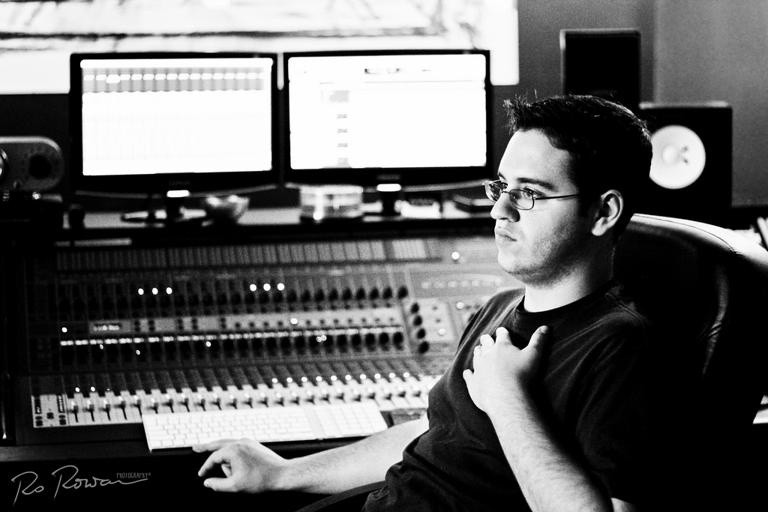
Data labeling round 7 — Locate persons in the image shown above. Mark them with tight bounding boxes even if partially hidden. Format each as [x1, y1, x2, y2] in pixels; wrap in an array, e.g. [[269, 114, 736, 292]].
[[188, 83, 684, 511]]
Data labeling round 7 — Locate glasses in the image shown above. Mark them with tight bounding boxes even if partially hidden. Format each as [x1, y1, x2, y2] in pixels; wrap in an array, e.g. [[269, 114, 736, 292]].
[[483, 179, 578, 210]]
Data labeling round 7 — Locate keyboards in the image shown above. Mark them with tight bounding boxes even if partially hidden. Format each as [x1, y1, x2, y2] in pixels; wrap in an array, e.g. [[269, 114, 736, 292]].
[[140, 400, 389, 453]]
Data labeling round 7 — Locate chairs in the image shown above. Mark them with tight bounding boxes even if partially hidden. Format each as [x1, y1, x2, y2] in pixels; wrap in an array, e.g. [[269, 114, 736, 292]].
[[292, 203, 767, 512]]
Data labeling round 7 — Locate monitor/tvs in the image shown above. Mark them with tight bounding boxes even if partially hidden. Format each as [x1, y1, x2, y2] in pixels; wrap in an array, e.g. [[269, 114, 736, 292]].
[[282, 49, 492, 218], [69, 52, 279, 224]]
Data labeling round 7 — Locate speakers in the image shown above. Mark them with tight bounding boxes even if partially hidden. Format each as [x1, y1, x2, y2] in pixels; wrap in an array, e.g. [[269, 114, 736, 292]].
[[559, 28, 642, 107], [635, 100, 734, 223]]
